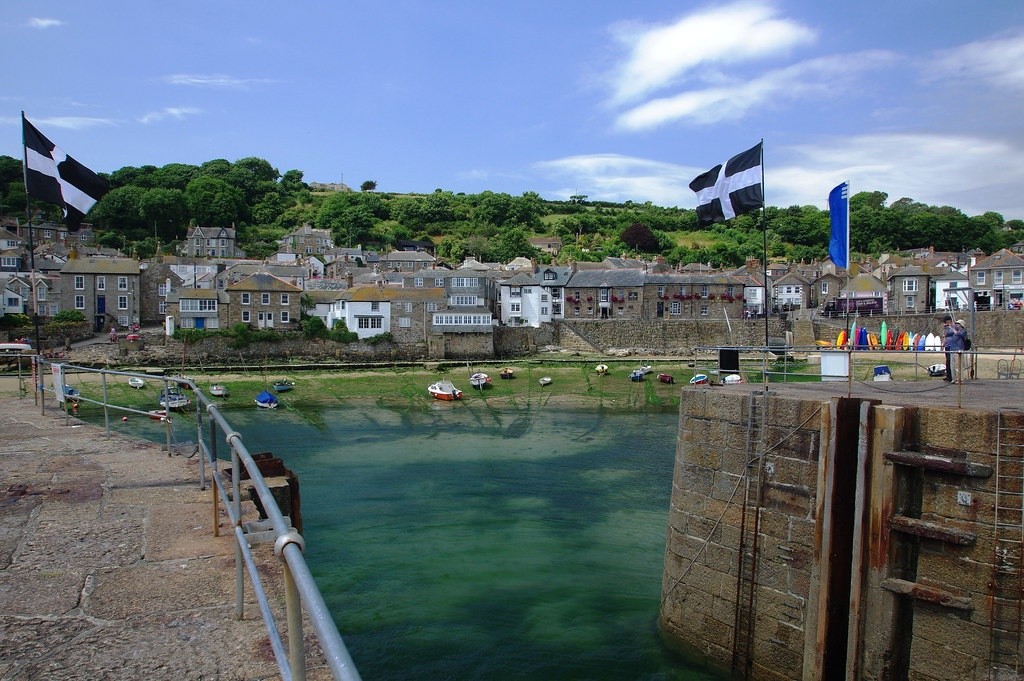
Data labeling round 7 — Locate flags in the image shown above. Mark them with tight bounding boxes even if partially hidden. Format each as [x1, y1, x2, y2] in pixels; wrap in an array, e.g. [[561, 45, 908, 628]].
[[826, 182, 848, 268], [688, 141, 765, 227], [23, 117, 109, 233]]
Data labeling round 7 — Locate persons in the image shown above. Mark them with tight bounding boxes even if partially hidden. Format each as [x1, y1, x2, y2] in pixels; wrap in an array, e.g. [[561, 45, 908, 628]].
[[947, 319, 969, 384], [941, 316, 955, 381]]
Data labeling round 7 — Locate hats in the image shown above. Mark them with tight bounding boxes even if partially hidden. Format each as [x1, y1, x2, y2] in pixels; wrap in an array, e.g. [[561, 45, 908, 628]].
[[954, 320, 966, 329]]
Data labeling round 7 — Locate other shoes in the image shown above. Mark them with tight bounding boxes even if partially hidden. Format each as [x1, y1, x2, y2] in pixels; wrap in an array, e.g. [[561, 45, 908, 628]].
[[955, 381, 963, 384], [950, 380, 956, 384], [943, 378, 951, 381]]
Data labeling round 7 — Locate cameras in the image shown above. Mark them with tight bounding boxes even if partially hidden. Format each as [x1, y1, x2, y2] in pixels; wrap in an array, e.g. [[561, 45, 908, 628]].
[[945, 324, 949, 326]]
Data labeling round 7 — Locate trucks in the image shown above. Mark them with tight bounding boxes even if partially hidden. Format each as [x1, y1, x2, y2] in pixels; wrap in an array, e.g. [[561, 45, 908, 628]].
[[822, 295, 884, 318]]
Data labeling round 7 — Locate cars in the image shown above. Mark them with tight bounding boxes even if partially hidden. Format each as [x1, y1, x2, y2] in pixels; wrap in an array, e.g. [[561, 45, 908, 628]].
[[0, 343, 31, 355]]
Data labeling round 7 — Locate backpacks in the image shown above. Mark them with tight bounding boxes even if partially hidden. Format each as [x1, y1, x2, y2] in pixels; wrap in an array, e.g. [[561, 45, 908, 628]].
[[961, 337, 971, 350]]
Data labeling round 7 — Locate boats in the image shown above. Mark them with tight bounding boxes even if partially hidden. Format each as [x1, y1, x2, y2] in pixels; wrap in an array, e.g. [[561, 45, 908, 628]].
[[174, 373, 195, 389], [640, 366, 652, 374], [272, 379, 295, 392], [594, 364, 608, 375], [209, 384, 228, 397], [428, 379, 463, 401], [62, 384, 82, 401], [659, 374, 676, 384], [720, 374, 745, 385], [631, 369, 644, 381], [539, 376, 551, 386], [469, 372, 488, 389], [59, 398, 78, 411], [255, 389, 278, 408], [159, 393, 188, 413], [500, 368, 514, 379], [873, 365, 893, 382], [927, 363, 946, 376], [148, 410, 167, 419], [128, 376, 144, 389], [689, 374, 707, 385]]
